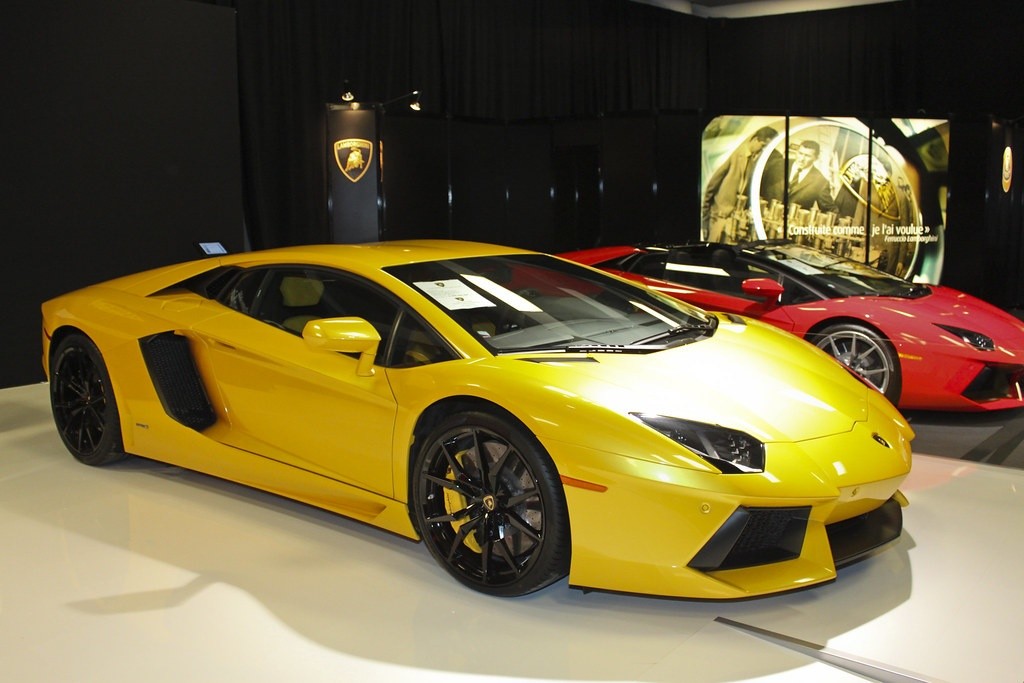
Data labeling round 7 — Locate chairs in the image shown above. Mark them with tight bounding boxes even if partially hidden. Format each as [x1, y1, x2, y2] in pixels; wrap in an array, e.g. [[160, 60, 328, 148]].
[[711, 250, 741, 293], [279, 278, 324, 334], [666, 252, 701, 288]]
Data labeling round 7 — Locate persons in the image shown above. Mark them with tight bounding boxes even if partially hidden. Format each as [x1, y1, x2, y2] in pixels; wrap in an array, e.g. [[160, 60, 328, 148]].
[[703, 124, 917, 278]]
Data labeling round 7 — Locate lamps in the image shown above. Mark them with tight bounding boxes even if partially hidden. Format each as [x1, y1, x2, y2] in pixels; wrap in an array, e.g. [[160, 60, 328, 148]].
[[374, 90, 422, 111], [340, 75, 354, 100]]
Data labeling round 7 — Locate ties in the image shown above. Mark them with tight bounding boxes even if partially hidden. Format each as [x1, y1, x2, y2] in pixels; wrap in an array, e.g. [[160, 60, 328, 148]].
[[788, 168, 802, 194]]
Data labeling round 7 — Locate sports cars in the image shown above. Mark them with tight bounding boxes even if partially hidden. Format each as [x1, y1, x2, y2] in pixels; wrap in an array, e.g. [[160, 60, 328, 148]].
[[42, 240, 915, 599], [483, 241, 1024, 412]]
